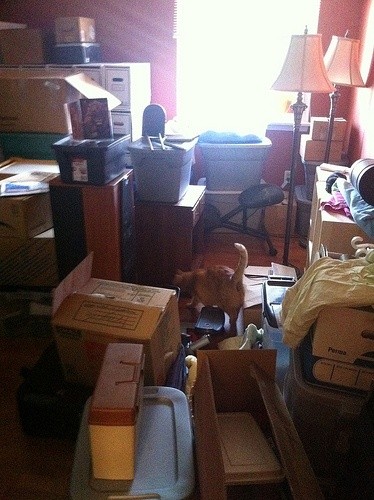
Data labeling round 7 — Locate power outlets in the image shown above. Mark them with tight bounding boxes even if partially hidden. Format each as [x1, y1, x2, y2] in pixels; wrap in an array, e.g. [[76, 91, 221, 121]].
[[284, 171, 291, 184]]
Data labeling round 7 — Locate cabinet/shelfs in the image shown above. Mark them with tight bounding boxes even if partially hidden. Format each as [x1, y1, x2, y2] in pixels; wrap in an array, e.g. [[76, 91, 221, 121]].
[[0, 62, 151, 141]]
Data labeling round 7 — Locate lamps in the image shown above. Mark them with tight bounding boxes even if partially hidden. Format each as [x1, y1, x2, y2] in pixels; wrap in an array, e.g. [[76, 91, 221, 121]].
[[324, 29, 366, 163], [271, 25, 337, 280]]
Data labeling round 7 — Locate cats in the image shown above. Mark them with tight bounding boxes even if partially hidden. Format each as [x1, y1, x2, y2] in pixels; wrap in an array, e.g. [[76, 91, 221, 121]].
[[168, 242, 248, 337]]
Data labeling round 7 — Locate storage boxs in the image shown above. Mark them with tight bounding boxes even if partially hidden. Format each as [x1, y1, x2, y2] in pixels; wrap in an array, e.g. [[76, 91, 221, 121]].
[[262, 275, 297, 379], [193, 350, 325, 500], [198, 177, 266, 233], [301, 157, 346, 201], [0, 70, 123, 136], [299, 134, 342, 161], [89, 343, 145, 480], [294, 185, 312, 248], [264, 191, 297, 238], [300, 252, 374, 398], [311, 199, 374, 266], [0, 134, 206, 390], [70, 386, 195, 500], [283, 348, 365, 478], [314, 166, 335, 190], [197, 136, 272, 191], [0, 16, 100, 63], [310, 182, 332, 241], [310, 117, 347, 141]]
